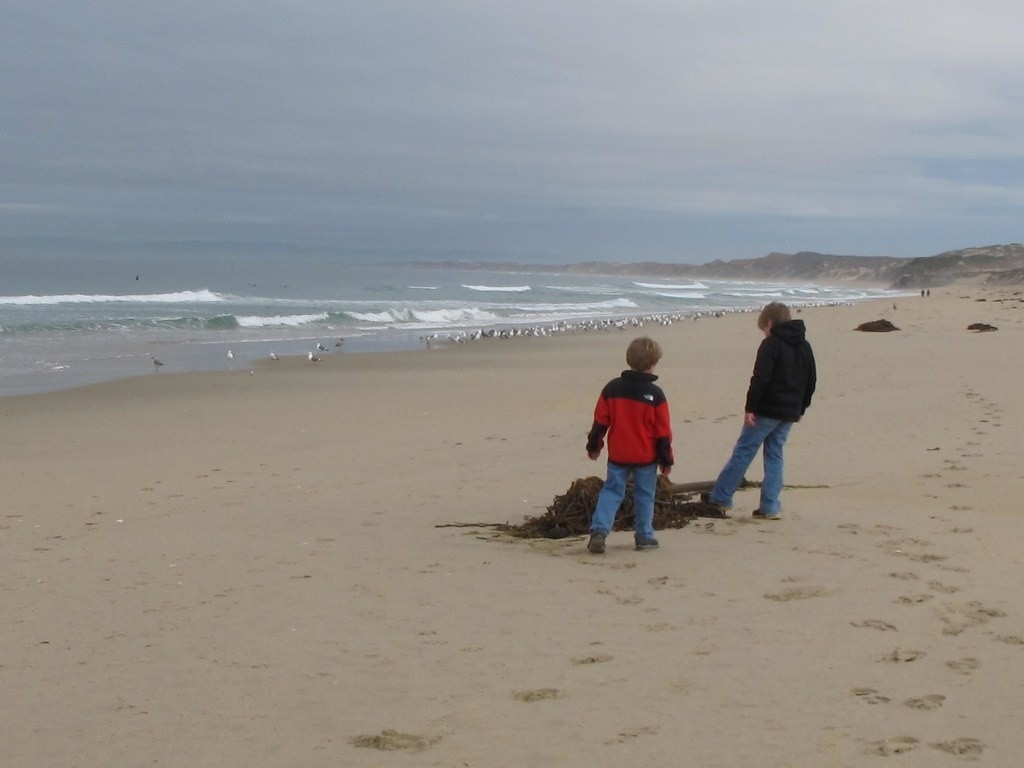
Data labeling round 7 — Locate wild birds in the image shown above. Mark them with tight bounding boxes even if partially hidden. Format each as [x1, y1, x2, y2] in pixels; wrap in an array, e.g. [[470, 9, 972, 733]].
[[151, 356, 165, 368], [268, 336, 347, 364], [225, 349, 234, 359], [410, 299, 855, 345]]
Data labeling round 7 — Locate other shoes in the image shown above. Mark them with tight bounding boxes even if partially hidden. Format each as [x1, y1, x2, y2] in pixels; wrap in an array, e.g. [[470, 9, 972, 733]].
[[634, 533, 659, 549], [753, 508, 780, 519], [586, 529, 606, 553], [695, 505, 726, 519]]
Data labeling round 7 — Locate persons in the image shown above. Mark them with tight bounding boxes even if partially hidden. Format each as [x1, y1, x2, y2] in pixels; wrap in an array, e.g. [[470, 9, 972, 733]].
[[700, 301, 817, 519], [586, 336, 674, 556]]
